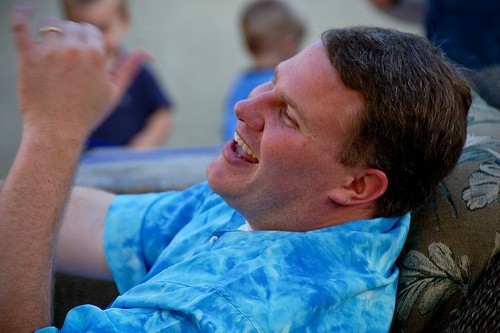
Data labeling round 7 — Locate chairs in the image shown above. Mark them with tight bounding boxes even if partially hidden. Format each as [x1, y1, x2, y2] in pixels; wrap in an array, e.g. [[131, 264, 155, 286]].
[[390, 84, 500, 333]]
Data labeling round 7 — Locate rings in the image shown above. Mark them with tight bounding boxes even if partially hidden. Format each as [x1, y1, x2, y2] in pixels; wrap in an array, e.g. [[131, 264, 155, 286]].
[[37, 27, 64, 33]]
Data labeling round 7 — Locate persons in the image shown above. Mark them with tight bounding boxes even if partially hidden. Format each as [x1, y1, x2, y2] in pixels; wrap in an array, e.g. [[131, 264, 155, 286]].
[[371, 0, 500, 168], [223, 0, 308, 144], [51, 0, 174, 154], [0, 0, 473, 333]]
